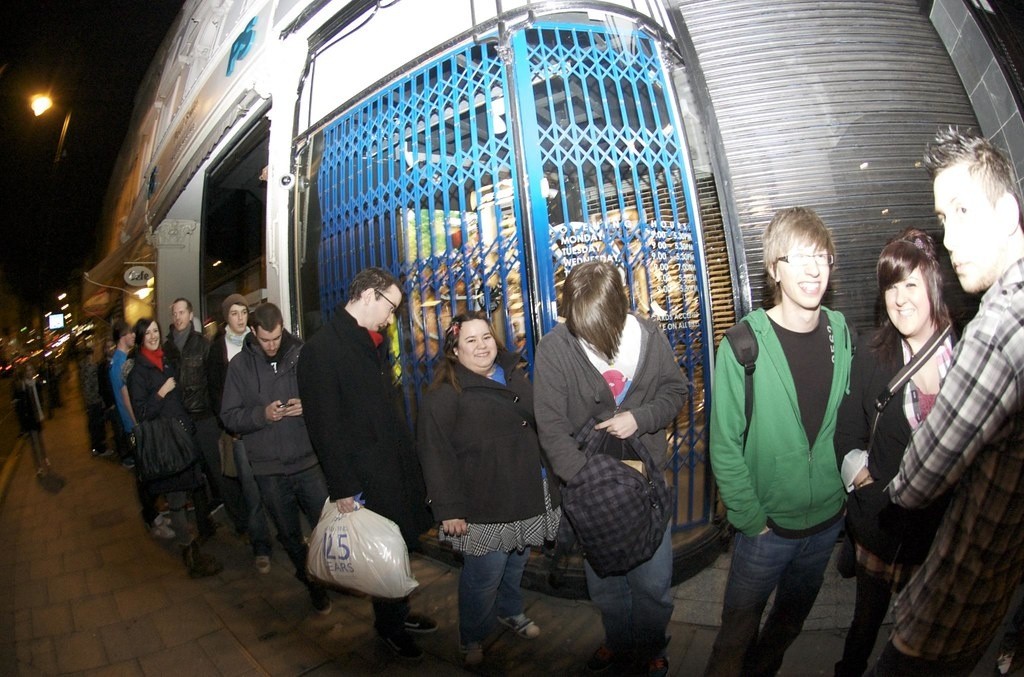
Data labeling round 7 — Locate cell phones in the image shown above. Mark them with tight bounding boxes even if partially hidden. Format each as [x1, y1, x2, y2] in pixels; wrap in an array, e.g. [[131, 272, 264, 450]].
[[280, 403, 289, 408]]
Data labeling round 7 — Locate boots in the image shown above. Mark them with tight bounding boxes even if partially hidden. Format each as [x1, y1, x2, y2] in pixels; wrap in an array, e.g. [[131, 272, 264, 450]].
[[178, 535, 223, 575]]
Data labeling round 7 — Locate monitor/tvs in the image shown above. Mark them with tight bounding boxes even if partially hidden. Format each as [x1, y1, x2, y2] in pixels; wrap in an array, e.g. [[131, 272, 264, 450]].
[[49, 314, 64, 330]]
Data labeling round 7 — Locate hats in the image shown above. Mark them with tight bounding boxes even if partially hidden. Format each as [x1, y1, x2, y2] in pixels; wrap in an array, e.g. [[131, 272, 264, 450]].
[[222, 293, 248, 322]]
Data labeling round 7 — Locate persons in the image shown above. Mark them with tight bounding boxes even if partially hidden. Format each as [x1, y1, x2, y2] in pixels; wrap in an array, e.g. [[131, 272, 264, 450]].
[[220, 303, 368, 615], [297, 267, 440, 653], [533, 259, 687, 675], [710, 207, 852, 675], [18, 363, 56, 479], [417, 311, 562, 667], [77, 124, 1024, 677], [126, 316, 226, 579]]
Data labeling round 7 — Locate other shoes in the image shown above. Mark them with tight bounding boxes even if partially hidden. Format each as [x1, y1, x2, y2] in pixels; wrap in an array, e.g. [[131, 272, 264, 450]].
[[257, 555, 271, 575], [184, 494, 195, 511], [343, 586, 366, 598], [404, 613, 439, 632], [91, 445, 114, 456], [497, 612, 541, 638], [154, 498, 170, 514], [307, 578, 333, 615], [459, 640, 482, 665], [145, 515, 176, 538], [121, 457, 135, 467], [380, 632, 425, 660]]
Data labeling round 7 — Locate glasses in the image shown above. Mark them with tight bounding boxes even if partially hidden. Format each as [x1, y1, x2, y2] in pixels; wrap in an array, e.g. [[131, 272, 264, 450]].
[[778, 253, 834, 266], [374, 289, 399, 314]]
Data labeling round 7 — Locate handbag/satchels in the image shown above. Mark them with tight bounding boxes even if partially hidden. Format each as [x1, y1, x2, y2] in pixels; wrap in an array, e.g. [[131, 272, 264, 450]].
[[306, 495, 419, 598], [558, 411, 674, 578], [134, 402, 196, 477]]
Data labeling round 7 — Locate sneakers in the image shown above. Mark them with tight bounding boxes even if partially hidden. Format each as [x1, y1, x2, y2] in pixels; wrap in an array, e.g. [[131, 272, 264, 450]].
[[586, 644, 620, 672], [648, 655, 669, 677]]
